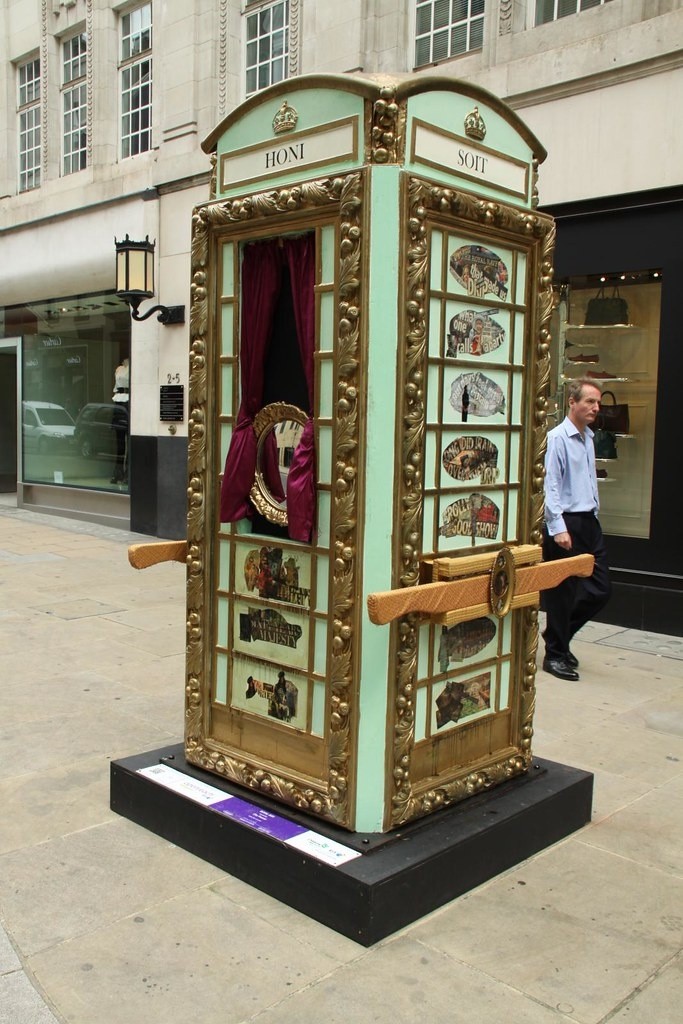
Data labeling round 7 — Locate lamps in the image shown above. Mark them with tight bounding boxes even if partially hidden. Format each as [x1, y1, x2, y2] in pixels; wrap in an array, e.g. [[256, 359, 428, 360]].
[[111, 233, 185, 327]]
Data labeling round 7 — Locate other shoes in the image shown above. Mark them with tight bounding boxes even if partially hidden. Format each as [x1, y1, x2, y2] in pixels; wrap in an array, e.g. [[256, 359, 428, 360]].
[[568, 353, 599, 364], [587, 371, 616, 378], [122, 480, 128, 486], [110, 477, 124, 484]]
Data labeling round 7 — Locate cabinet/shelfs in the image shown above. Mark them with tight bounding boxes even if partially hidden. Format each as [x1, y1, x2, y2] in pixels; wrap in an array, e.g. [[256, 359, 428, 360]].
[[566, 320, 638, 483]]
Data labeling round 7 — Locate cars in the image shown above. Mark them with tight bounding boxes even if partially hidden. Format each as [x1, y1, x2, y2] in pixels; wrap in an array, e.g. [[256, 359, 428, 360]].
[[22, 400, 76, 456], [73, 402, 130, 461]]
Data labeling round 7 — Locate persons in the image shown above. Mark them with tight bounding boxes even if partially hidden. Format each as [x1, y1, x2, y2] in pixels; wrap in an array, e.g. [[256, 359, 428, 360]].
[[111, 359, 128, 485], [539, 376, 610, 680]]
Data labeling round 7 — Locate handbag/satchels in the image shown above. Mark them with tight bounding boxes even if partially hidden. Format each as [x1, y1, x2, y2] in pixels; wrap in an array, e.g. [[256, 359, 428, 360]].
[[587, 391, 630, 434], [584, 286, 628, 326], [592, 429, 618, 459]]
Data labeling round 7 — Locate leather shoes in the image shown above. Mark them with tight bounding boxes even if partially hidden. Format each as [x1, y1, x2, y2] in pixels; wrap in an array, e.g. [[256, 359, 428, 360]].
[[565, 649, 578, 667], [543, 657, 579, 679]]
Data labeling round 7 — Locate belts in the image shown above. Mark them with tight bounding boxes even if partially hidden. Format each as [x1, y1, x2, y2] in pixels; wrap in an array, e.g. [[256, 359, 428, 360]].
[[116, 387, 129, 394]]
